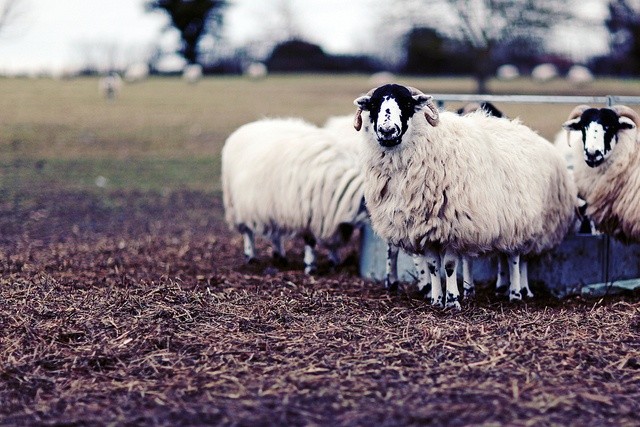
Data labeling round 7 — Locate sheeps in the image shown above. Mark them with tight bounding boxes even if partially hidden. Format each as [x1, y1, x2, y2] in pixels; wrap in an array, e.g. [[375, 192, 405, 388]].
[[383, 98, 509, 300], [219, 97, 444, 276], [352, 81, 575, 310], [553, 103, 640, 248]]
